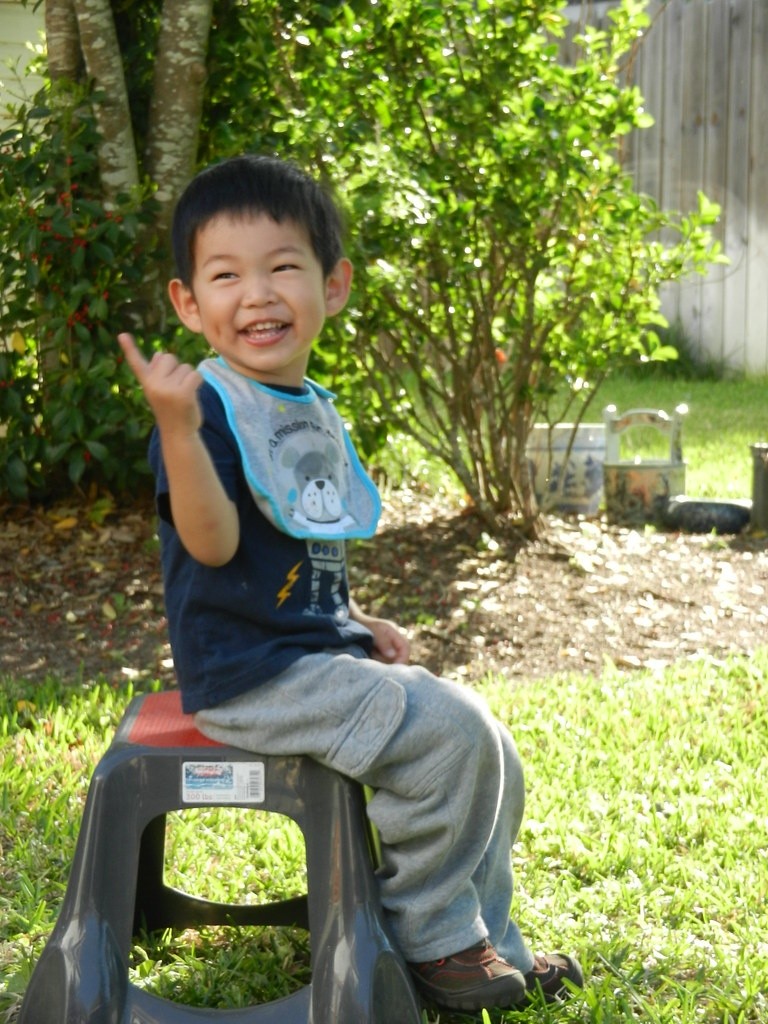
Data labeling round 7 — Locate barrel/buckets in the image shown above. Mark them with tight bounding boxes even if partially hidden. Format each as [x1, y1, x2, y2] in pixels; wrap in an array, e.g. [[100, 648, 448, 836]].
[[601, 402, 688, 526]]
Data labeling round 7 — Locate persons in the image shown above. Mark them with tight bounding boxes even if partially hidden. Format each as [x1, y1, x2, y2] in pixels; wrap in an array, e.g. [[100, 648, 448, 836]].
[[117, 159, 583, 1012]]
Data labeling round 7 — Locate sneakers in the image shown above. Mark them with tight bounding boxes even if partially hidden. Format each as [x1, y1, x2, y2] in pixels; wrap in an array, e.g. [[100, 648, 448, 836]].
[[509, 954, 583, 1008], [408, 936, 526, 1009]]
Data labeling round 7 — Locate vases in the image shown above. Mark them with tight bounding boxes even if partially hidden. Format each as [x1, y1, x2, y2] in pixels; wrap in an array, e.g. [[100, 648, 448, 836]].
[[531, 425, 607, 510]]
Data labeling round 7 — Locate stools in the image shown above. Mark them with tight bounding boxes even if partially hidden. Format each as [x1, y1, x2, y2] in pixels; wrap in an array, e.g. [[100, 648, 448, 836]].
[[16, 691, 427, 1023]]
